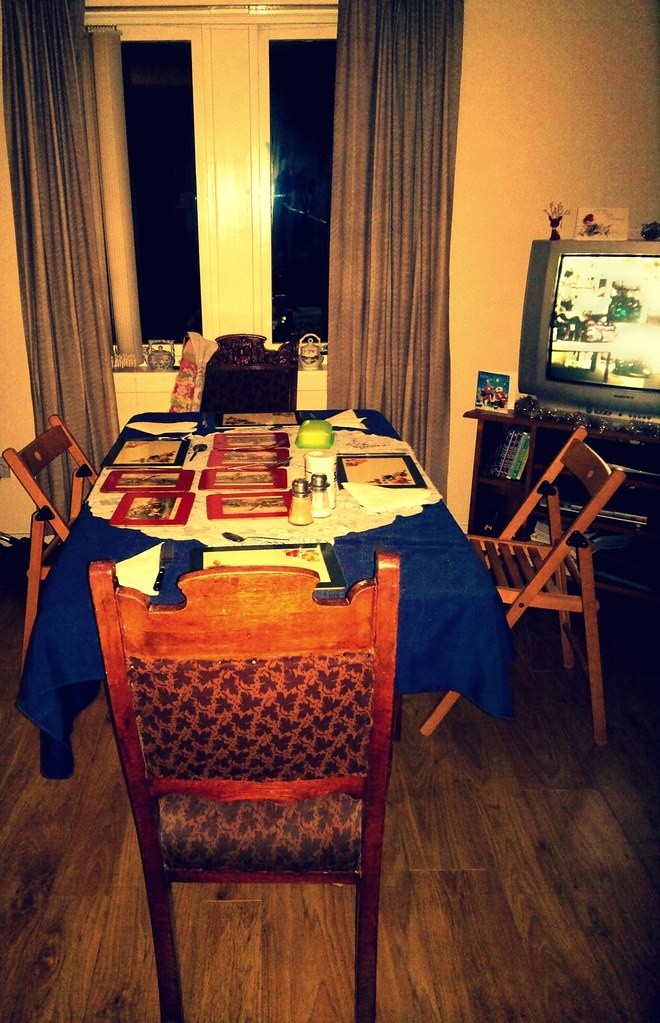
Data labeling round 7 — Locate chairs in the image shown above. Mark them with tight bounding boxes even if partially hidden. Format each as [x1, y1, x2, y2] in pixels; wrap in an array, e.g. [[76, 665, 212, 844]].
[[89, 550, 402, 1023], [420, 423, 624, 744], [184, 333, 298, 414], [2, 413, 98, 676]]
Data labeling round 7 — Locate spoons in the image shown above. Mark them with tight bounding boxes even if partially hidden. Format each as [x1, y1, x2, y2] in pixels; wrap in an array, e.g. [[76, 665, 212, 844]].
[[189, 444, 207, 462], [218, 439, 287, 449], [216, 424, 284, 430], [227, 456, 293, 469], [223, 532, 290, 542]]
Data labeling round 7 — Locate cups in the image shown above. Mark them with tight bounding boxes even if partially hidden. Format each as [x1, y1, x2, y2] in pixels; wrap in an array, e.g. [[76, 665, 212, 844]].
[[304, 449, 337, 509]]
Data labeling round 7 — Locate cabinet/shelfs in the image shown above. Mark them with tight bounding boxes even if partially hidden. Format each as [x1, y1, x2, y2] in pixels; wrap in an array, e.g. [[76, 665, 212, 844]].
[[465, 410, 660, 622]]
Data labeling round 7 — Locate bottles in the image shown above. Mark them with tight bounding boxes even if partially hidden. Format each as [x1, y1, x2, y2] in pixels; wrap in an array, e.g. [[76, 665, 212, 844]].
[[288, 475, 331, 525]]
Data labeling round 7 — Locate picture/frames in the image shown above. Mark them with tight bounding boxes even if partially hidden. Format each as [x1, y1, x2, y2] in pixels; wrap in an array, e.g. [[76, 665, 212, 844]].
[[207, 448, 289, 468], [194, 542, 348, 591], [198, 468, 287, 491], [335, 455, 427, 489], [101, 469, 195, 493], [205, 492, 292, 520], [109, 490, 195, 527], [101, 438, 191, 468], [213, 432, 291, 448]]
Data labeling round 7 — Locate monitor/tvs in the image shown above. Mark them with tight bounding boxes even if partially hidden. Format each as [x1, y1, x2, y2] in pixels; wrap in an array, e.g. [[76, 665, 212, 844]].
[[519, 238, 660, 427]]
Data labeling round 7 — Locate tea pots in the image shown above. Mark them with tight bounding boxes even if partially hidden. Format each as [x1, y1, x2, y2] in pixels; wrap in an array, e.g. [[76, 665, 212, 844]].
[[143, 340, 176, 370], [298, 333, 324, 370]]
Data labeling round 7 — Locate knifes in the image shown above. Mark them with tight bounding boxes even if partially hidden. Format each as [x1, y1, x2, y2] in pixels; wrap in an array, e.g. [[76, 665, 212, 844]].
[[336, 453, 406, 456]]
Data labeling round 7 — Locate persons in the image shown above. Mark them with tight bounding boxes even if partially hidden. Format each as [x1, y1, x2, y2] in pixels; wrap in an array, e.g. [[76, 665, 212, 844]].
[[484, 380, 493, 404], [578, 214, 599, 238], [560, 269, 579, 313], [492, 386, 507, 411]]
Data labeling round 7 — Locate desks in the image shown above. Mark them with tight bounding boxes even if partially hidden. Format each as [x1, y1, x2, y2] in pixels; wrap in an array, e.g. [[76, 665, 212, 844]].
[[14, 410, 518, 781]]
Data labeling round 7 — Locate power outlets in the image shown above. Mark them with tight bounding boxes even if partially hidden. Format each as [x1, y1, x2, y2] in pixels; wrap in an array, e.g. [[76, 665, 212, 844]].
[[0, 457, 11, 479]]
